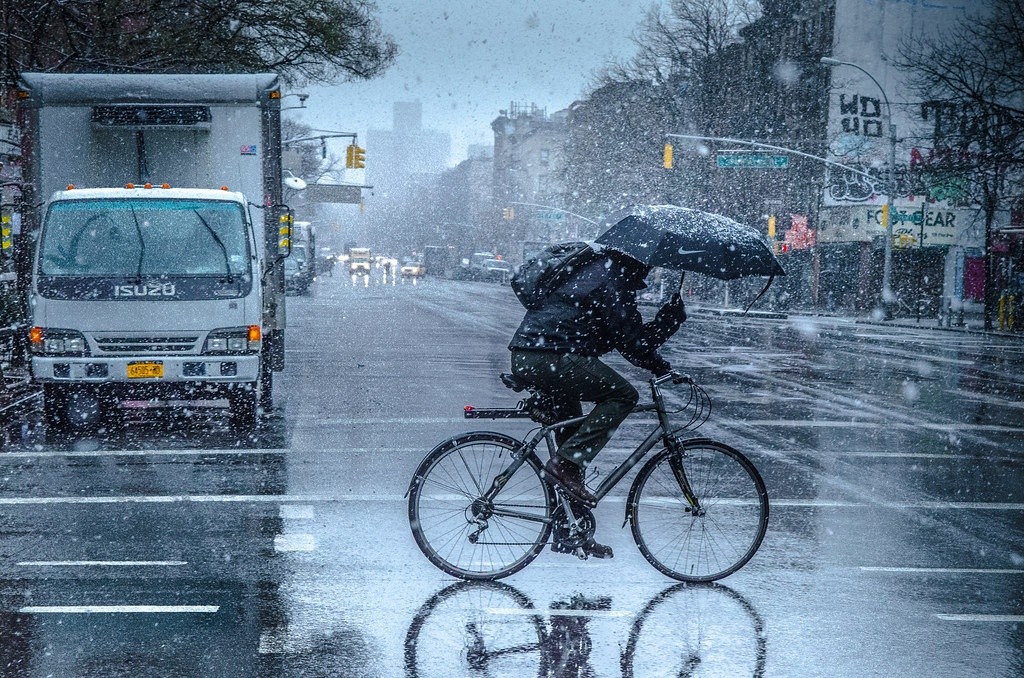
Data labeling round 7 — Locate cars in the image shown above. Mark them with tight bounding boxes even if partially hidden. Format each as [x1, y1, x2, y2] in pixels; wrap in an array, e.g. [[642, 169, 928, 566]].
[[375, 243, 522, 287], [283, 219, 351, 297], [401, 261, 425, 278]]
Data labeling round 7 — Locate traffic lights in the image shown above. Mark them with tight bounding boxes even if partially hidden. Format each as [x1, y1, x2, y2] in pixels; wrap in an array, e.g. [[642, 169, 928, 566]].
[[503, 207, 509, 219], [354, 145, 366, 168]]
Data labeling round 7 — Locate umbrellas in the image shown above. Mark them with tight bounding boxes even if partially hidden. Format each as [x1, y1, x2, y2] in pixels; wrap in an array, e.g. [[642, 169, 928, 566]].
[[594, 206, 786, 303]]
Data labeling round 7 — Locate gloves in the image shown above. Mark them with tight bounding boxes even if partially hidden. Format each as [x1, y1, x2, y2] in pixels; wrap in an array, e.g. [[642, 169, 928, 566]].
[[656, 293, 687, 324], [651, 360, 672, 378]]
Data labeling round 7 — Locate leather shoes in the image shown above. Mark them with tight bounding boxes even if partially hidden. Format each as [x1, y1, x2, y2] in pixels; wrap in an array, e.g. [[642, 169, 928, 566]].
[[540, 454, 597, 508], [551, 528, 613, 559]]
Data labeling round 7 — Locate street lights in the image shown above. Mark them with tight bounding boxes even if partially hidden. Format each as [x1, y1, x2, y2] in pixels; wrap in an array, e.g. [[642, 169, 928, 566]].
[[821, 55, 895, 321]]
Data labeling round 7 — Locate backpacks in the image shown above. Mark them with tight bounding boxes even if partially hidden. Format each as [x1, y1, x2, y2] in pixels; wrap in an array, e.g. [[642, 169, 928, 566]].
[[510, 242, 606, 310]]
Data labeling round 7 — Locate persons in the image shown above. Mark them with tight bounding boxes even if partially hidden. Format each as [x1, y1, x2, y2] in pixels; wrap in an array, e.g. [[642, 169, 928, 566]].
[[385, 262, 390, 274], [326, 256, 334, 275], [996, 250, 1019, 332], [508, 245, 687, 559]]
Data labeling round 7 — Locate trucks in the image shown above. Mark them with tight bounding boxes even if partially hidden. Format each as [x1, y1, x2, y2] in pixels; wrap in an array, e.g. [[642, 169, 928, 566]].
[[0, 71, 294, 432], [349, 246, 373, 274]]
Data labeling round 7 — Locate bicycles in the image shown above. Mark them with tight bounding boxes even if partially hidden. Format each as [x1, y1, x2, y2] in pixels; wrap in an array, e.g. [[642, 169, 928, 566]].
[[403, 365, 769, 583], [883, 279, 939, 320]]
